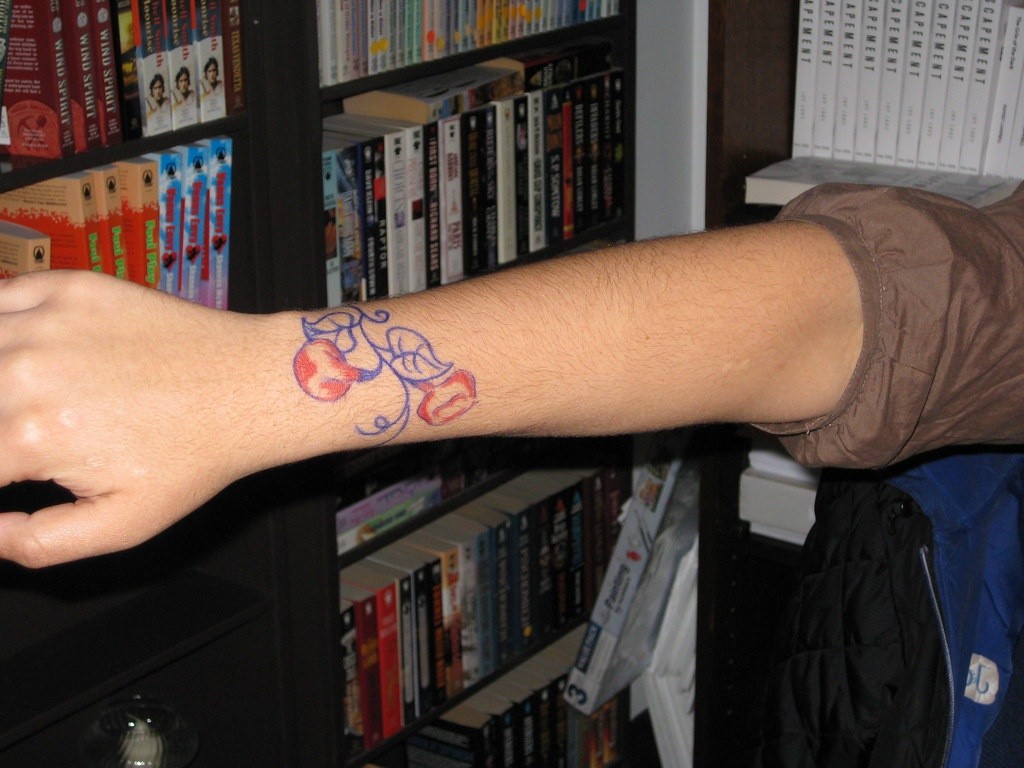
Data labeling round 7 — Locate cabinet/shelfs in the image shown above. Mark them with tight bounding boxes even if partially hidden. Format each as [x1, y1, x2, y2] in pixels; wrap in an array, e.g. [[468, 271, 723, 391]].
[[0, 0, 818, 768]]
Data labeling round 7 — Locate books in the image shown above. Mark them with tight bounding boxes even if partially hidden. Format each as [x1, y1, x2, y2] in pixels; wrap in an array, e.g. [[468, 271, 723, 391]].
[[0, 1, 1024, 768]]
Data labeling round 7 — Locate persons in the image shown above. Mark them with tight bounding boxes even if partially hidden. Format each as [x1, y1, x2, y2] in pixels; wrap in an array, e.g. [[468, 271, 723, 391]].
[[202, 57, 223, 95], [142, 73, 168, 112], [0, 181, 1024, 567], [171, 67, 195, 103]]
